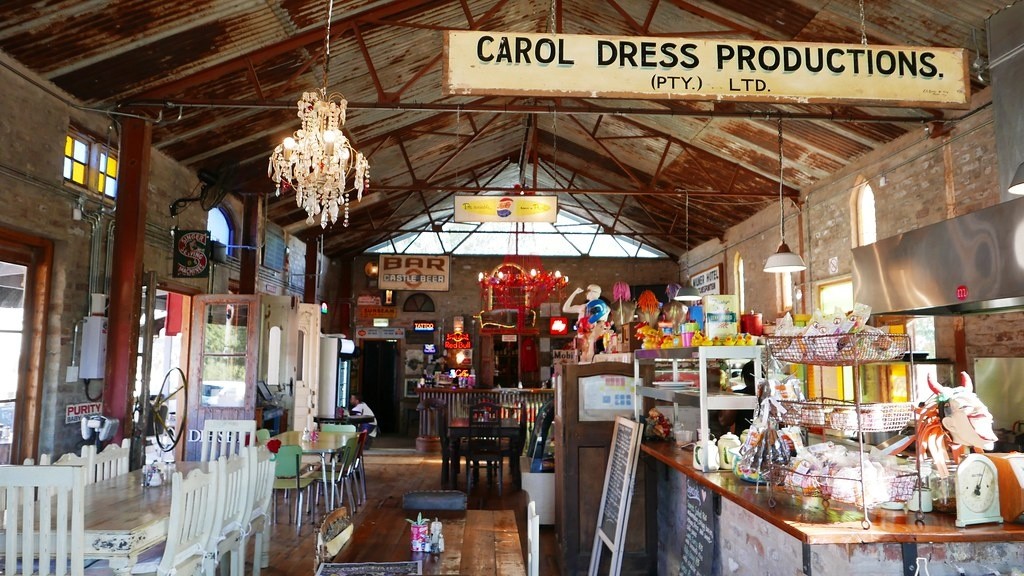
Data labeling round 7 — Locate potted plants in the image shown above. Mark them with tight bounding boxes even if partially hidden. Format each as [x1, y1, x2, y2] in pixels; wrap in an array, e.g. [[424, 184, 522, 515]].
[[404, 512, 430, 552]]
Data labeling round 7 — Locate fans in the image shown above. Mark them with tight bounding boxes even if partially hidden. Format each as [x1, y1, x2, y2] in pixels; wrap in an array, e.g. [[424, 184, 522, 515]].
[[169, 156, 241, 219]]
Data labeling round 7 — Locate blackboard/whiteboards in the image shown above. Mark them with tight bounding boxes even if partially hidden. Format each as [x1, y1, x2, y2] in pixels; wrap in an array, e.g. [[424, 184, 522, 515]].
[[596, 416, 639, 553]]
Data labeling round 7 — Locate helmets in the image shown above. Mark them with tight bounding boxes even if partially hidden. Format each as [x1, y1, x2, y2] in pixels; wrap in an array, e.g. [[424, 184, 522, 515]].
[[586, 299, 608, 324]]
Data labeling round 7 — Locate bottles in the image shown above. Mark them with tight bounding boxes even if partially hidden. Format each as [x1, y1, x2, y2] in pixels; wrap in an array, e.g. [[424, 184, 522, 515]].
[[438, 533, 445, 552], [424, 535, 430, 552], [430, 518, 442, 552]]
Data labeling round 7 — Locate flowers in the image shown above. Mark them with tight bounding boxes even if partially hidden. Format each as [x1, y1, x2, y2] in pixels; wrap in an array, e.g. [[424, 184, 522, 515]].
[[267, 439, 281, 461], [245, 435, 258, 445]]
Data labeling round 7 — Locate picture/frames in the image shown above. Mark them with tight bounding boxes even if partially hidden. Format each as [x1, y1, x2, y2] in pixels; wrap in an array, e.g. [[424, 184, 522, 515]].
[[404, 378, 419, 398]]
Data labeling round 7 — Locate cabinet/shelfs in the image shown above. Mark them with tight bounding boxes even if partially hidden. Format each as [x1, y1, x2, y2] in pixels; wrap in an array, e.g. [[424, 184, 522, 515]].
[[765, 332, 926, 530], [633, 345, 762, 474]]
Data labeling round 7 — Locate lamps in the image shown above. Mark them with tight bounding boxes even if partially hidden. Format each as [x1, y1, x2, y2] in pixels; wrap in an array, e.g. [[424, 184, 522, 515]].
[[673, 194, 702, 301], [763, 119, 807, 272], [267, 0, 371, 228], [477, 184, 570, 316], [1008, 162, 1024, 196]]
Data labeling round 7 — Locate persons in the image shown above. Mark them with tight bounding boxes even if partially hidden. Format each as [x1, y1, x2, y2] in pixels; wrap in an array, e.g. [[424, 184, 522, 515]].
[[563, 283, 611, 339], [662, 284, 689, 335], [609, 281, 637, 327], [349, 393, 378, 451], [635, 291, 660, 328]]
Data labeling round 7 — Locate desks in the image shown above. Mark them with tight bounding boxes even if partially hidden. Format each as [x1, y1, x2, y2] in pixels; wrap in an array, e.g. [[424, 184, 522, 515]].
[[327, 509, 526, 576], [0, 460, 272, 576], [257, 430, 361, 516], [447, 417, 521, 493], [313, 414, 375, 431]]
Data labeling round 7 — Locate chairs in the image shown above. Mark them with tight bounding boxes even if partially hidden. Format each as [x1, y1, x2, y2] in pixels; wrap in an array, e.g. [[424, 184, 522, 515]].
[[295, 433, 360, 523], [271, 445, 316, 535], [205, 447, 251, 576], [313, 424, 357, 507], [89, 437, 131, 484], [256, 429, 289, 499], [313, 507, 349, 573], [438, 396, 528, 497], [0, 464, 86, 576], [131, 461, 219, 576], [86, 461, 219, 576], [219, 444, 276, 576], [200, 419, 258, 462], [527, 501, 540, 576], [316, 429, 368, 507], [403, 489, 468, 510], [37, 445, 90, 499]]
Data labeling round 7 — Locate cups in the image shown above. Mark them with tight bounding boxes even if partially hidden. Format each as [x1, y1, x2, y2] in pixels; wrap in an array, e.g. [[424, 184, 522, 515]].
[[163, 460, 177, 483], [685, 323, 698, 332], [693, 438, 720, 470], [718, 432, 741, 469], [681, 331, 695, 347]]
